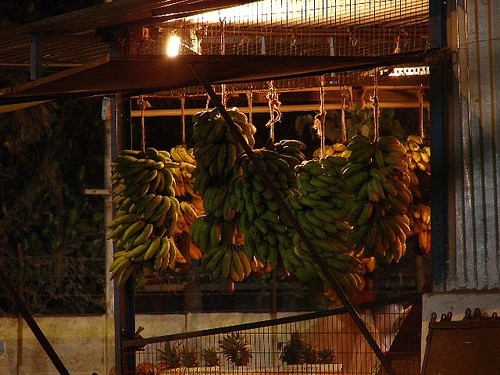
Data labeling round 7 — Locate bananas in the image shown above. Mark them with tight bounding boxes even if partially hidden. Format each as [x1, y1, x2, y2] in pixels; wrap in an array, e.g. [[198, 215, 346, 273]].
[[108, 104, 431, 289]]
